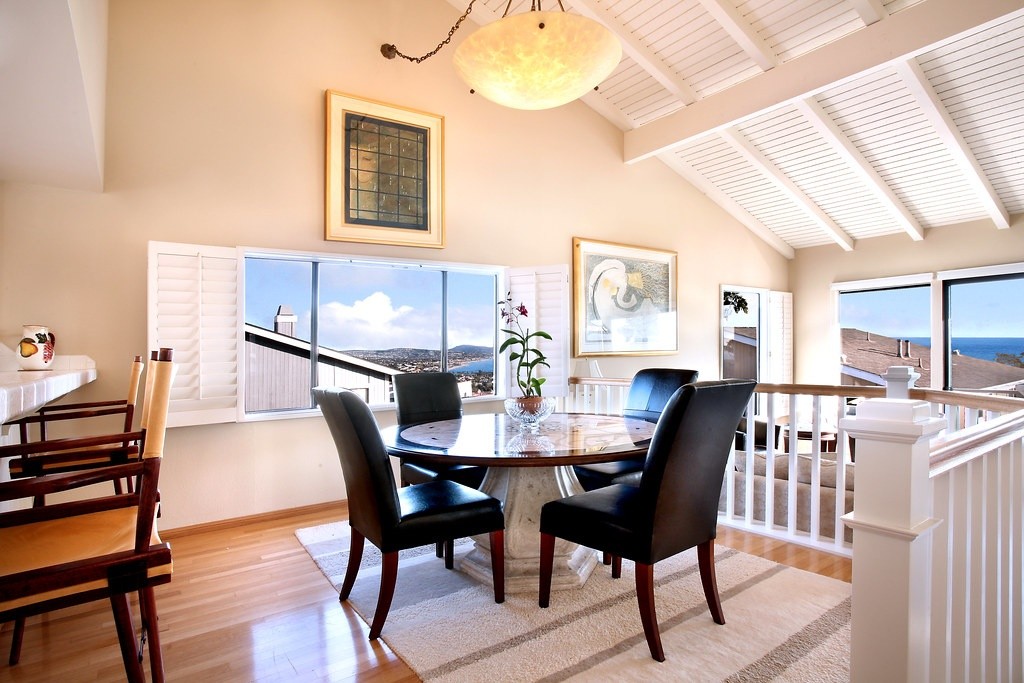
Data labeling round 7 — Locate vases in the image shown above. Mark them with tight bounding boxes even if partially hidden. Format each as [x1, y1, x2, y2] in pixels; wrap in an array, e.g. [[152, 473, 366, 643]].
[[504, 397, 556, 426]]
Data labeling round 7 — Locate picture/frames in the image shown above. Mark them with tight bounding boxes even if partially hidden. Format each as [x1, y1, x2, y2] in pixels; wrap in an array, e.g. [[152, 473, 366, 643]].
[[571, 236, 680, 359], [324, 87, 446, 250]]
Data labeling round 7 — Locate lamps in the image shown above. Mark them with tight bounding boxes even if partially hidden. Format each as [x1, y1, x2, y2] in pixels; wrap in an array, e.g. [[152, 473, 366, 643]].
[[380, 0, 621, 110]]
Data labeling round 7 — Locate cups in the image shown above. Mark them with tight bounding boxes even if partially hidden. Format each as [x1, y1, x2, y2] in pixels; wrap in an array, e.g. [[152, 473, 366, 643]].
[[16, 325, 56, 370]]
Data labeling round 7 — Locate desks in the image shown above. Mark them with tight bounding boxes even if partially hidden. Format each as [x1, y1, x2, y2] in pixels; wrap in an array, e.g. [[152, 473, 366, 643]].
[[0, 367, 97, 513], [379, 411, 656, 594]]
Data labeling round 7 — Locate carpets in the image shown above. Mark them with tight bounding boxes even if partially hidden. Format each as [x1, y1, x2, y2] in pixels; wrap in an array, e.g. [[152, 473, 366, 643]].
[[295, 520, 852, 683]]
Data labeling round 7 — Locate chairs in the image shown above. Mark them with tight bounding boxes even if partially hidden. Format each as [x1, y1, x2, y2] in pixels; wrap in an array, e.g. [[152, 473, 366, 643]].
[[392, 372, 488, 559], [0, 351, 163, 520], [312, 384, 506, 641], [539, 378, 757, 663], [2, 355, 140, 494], [0, 347, 175, 683], [574, 368, 699, 580]]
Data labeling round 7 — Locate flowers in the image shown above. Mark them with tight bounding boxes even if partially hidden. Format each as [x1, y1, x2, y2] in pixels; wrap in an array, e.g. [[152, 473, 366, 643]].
[[497, 291, 552, 398]]
